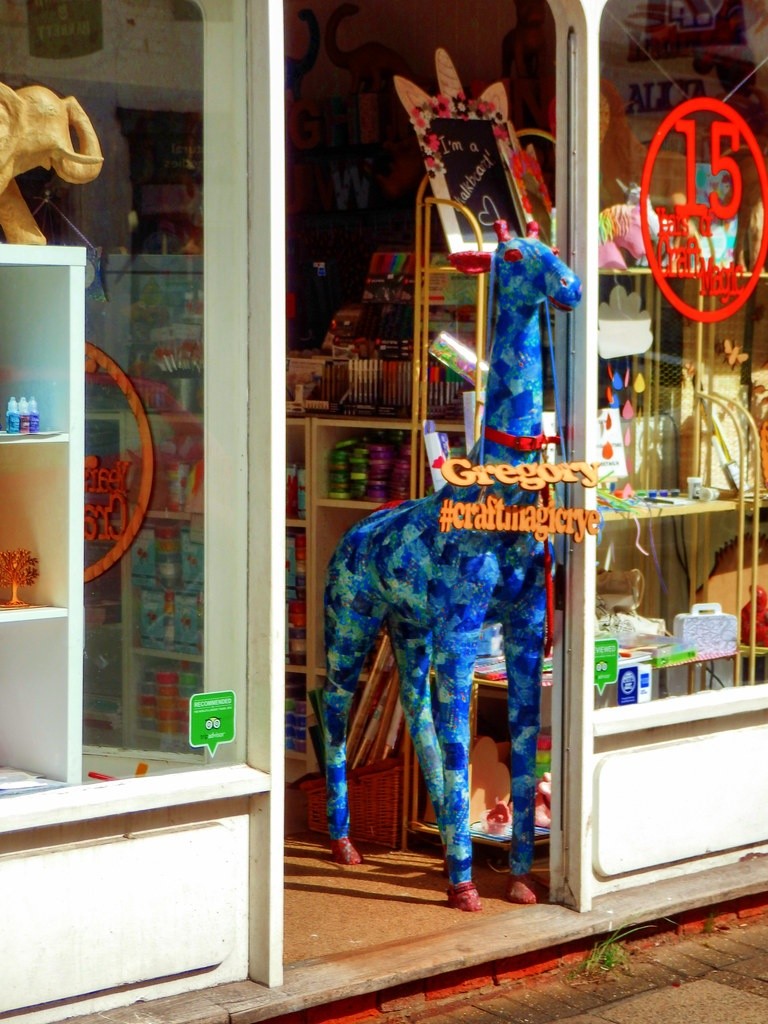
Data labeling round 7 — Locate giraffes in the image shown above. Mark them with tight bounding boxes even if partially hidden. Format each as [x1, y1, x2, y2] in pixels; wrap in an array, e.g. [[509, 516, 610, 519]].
[[319, 220, 586, 915]]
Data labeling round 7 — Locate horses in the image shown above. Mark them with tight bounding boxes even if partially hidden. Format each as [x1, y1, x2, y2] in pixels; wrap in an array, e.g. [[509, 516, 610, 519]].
[[597, 204, 645, 270]]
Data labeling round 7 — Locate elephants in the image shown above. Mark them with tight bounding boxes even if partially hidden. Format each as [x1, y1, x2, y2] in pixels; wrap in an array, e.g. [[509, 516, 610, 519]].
[[0, 81, 104, 246]]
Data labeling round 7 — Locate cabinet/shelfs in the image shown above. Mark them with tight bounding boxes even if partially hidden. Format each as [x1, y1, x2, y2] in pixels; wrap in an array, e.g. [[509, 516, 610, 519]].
[[0, 244, 87, 786], [84, 129, 768, 852]]
